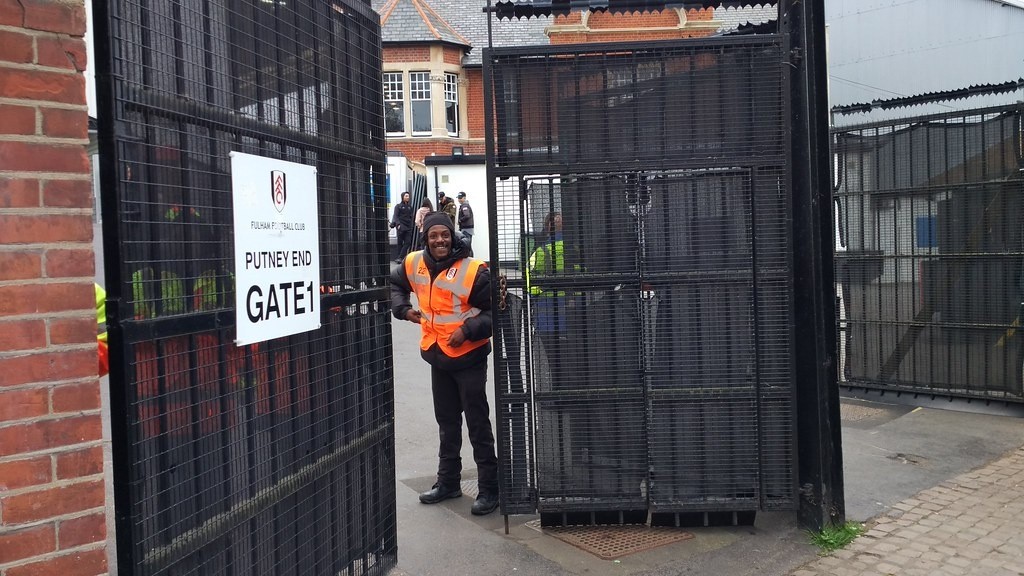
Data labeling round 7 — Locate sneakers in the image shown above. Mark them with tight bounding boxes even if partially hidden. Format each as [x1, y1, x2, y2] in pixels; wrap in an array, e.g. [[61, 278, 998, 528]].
[[419, 483, 462, 504], [471, 492, 499, 515]]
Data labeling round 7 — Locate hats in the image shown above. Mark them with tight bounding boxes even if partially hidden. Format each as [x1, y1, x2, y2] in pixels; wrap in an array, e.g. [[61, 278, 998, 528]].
[[437, 192, 445, 197], [456, 192, 466, 198], [423, 211, 455, 241]]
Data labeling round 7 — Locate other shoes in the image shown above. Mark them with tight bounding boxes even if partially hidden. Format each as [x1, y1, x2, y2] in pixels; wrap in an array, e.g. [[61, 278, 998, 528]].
[[395, 258, 402, 264]]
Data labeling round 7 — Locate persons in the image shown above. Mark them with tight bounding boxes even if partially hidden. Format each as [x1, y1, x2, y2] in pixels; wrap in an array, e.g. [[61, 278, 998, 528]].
[[97, 200, 348, 547], [389, 211, 499, 515], [389, 189, 476, 264], [525, 207, 589, 390]]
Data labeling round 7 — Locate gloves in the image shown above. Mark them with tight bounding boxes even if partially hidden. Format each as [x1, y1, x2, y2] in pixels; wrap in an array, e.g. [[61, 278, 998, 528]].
[[390, 222, 396, 228]]
